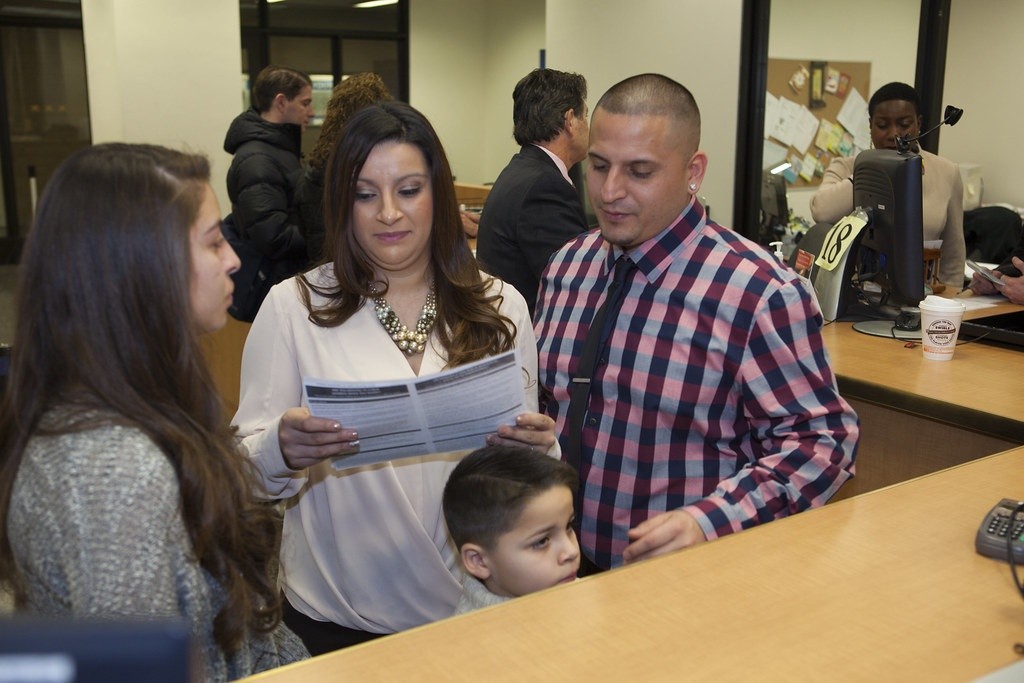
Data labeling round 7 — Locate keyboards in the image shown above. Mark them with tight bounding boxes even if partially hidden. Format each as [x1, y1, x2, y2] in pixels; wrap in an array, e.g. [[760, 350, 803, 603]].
[[960, 322, 1024, 344]]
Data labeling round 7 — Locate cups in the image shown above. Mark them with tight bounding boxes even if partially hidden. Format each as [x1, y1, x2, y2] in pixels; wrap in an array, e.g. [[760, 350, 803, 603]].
[[919, 300, 966, 360]]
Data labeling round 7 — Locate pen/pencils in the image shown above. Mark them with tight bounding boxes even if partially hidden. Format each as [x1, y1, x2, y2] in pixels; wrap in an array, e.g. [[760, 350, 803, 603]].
[[956, 280, 981, 295]]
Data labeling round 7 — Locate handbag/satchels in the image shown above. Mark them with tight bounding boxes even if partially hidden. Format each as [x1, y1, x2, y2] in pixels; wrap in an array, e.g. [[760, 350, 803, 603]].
[[219, 222, 291, 325]]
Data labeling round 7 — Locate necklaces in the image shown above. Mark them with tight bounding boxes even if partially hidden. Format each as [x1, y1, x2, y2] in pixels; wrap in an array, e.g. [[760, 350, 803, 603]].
[[366, 279, 438, 356]]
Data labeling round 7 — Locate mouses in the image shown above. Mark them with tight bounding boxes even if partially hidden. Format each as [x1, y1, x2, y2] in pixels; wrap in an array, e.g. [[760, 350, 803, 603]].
[[894, 312, 923, 332]]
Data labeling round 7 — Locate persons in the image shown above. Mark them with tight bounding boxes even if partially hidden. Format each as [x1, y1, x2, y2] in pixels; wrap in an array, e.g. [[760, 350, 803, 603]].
[[1, 140, 312, 683], [229, 99, 563, 655], [970, 240, 1024, 305], [441, 444, 582, 618], [531, 72, 863, 578], [223, 66, 590, 324], [808, 81, 968, 293]]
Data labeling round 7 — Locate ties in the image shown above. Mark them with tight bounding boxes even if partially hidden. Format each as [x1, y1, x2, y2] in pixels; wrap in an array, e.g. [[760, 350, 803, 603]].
[[561, 257, 637, 470]]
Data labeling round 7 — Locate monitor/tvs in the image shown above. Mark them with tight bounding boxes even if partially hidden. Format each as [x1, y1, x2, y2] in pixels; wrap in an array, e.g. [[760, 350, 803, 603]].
[[814, 150, 925, 323]]
